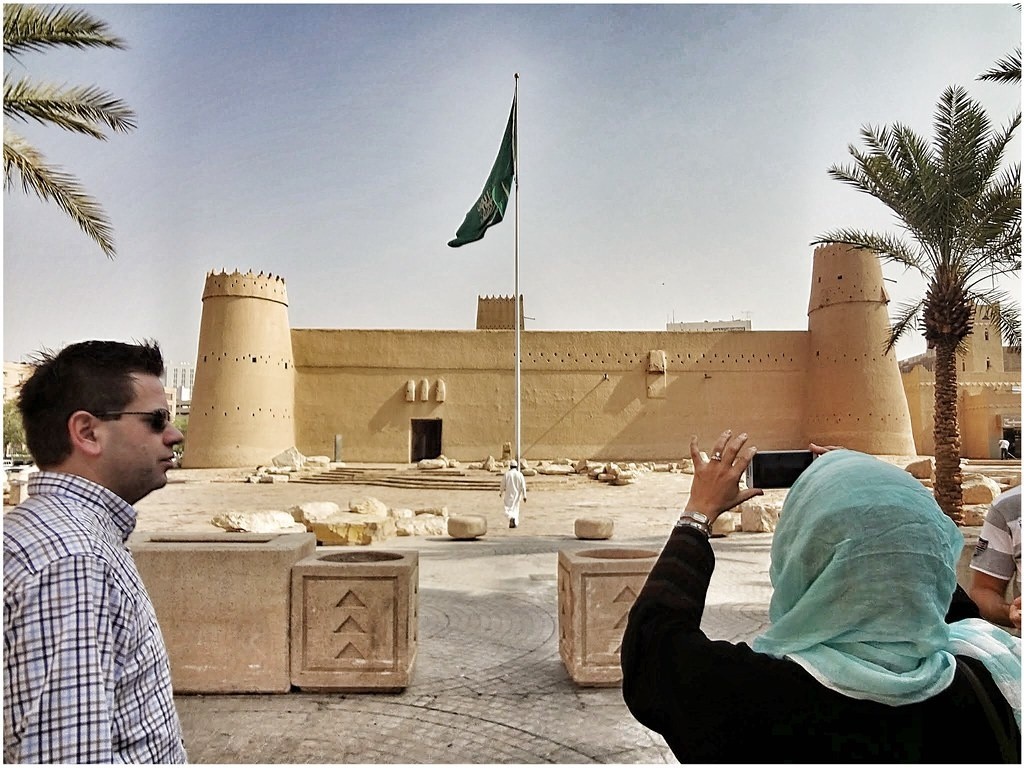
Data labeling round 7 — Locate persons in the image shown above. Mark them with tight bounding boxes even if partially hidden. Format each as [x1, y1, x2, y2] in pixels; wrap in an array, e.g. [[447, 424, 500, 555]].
[[972, 492, 1021, 631], [499, 458, 529, 531], [618, 426, 1021, 763], [997, 437, 1013, 458], [3, 341, 194, 765]]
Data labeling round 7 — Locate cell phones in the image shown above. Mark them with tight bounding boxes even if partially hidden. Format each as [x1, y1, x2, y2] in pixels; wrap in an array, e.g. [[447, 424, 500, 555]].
[[746, 450, 819, 489]]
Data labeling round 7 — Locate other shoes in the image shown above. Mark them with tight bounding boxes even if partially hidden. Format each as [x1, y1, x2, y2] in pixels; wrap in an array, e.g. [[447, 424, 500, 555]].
[[509, 518, 515, 528]]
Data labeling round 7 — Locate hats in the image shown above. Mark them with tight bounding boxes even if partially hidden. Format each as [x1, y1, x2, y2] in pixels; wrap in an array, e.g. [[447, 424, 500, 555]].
[[510, 460, 517, 466]]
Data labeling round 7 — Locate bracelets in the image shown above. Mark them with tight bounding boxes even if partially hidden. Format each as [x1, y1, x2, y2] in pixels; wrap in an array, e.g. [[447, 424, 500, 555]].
[[675, 520, 715, 537]]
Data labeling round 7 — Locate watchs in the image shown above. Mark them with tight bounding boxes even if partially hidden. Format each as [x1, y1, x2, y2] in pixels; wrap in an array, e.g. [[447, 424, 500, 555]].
[[679, 511, 713, 534]]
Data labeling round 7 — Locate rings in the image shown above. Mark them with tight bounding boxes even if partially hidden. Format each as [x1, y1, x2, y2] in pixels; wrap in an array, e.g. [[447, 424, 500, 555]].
[[711, 449, 724, 462]]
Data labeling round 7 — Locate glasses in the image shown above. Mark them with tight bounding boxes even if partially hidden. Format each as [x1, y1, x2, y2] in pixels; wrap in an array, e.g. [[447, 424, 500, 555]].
[[97, 409, 170, 433]]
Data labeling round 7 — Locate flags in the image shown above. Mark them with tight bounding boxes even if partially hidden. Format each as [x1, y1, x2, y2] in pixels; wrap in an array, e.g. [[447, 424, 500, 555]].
[[446, 82, 519, 250]]
